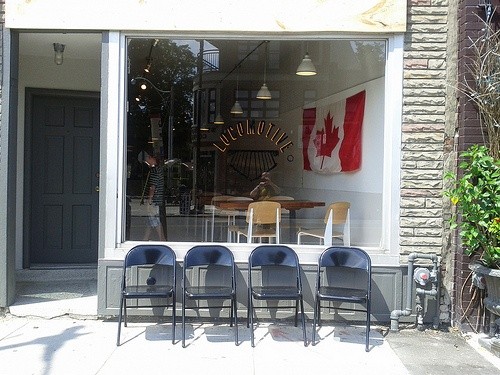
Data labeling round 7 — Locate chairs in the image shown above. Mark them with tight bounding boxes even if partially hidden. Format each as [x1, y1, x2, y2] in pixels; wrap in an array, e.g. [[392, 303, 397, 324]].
[[115, 244, 176, 346], [296, 202, 352, 248], [247, 245, 308, 346], [181, 246, 239, 348], [203, 194, 293, 245], [311, 246, 372, 352]]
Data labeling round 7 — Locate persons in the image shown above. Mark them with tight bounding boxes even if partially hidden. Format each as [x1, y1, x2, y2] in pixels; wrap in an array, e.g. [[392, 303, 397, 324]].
[[250, 172, 282, 243], [142, 149, 167, 241]]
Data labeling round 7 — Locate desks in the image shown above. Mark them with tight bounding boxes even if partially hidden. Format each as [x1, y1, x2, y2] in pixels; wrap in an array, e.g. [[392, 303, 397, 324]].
[[219, 198, 326, 243]]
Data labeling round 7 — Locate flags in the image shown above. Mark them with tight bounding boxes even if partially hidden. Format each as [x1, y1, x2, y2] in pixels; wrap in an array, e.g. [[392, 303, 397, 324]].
[[302, 89, 366, 175]]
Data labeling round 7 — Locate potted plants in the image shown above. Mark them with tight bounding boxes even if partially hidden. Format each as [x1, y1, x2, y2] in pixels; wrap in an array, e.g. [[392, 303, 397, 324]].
[[442, 143, 500, 316]]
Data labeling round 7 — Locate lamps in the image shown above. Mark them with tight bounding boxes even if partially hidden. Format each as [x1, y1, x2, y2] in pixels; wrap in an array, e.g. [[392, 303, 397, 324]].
[[255, 40, 273, 100], [295, 40, 318, 77], [135, 92, 144, 101], [140, 76, 147, 90], [54, 43, 65, 66], [229, 75, 244, 114], [144, 56, 152, 73], [151, 38, 159, 48], [214, 112, 224, 124], [198, 122, 210, 131]]
